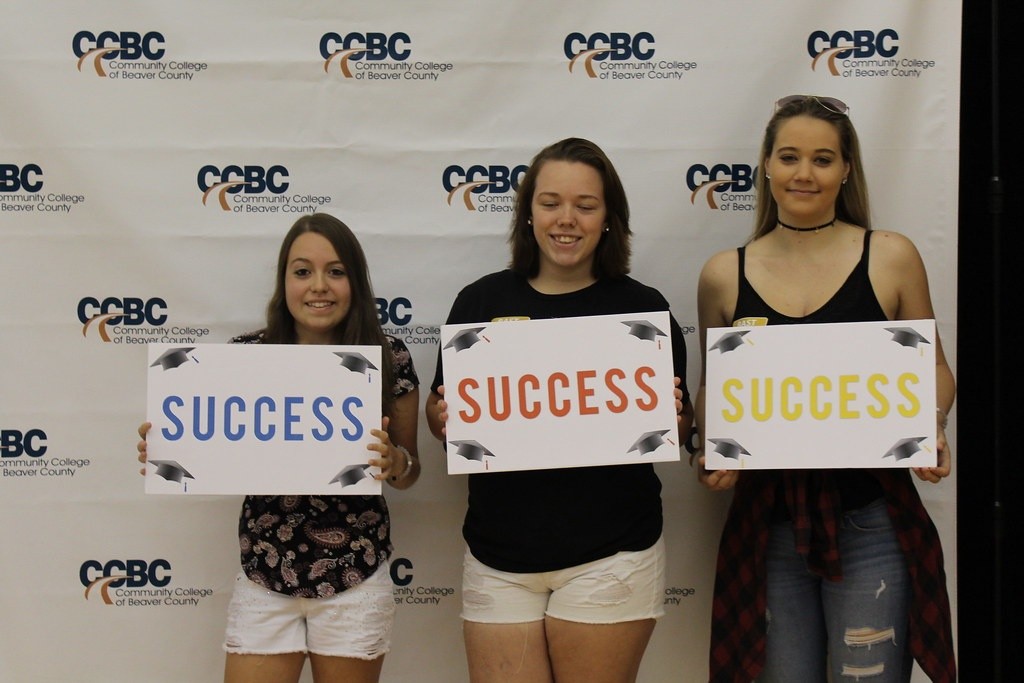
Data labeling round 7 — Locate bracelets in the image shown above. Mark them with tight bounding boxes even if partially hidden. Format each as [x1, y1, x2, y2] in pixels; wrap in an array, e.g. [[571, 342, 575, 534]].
[[386, 443, 413, 484], [935, 406, 948, 430]]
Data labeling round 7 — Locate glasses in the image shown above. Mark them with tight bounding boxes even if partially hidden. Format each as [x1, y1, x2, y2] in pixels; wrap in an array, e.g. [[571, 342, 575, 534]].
[[774, 94, 850, 121]]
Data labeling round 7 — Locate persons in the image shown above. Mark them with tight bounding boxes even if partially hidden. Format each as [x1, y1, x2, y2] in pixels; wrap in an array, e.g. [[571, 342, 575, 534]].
[[696, 92, 962, 683], [137, 213, 420, 682], [425, 136, 701, 682]]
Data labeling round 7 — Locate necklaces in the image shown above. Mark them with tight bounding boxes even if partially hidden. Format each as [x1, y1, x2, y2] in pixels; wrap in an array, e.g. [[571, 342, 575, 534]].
[[774, 214, 838, 235]]
